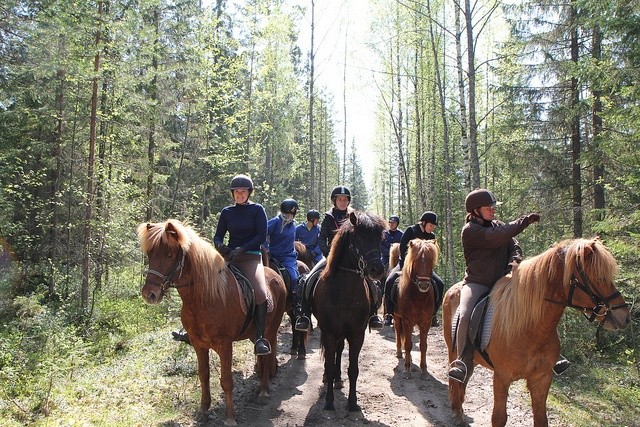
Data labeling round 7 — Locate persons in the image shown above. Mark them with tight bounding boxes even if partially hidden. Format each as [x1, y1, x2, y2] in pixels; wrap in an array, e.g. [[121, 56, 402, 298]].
[[378, 216, 404, 306], [295, 186, 384, 332], [384, 212, 444, 326], [295, 209, 323, 260], [449, 188, 572, 382], [171, 175, 272, 355], [261, 198, 299, 314]]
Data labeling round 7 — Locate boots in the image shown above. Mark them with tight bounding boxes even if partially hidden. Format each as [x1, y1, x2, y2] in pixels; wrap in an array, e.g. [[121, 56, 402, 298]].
[[295, 299, 312, 329], [432, 317, 440, 327], [254, 302, 268, 354], [170, 331, 192, 344], [369, 302, 383, 328], [385, 296, 394, 326]]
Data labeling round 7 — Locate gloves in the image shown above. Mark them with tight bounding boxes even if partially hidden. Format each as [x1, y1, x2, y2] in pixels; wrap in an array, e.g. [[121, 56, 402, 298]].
[[218, 245, 231, 254], [529, 214, 541, 223], [229, 246, 245, 257]]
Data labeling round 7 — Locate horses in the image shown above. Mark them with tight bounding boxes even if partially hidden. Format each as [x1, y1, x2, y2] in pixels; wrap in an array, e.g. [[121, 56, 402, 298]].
[[305, 211, 390, 411], [260, 245, 310, 370], [384, 239, 442, 380], [294, 241, 315, 332], [442, 236, 631, 427], [137, 219, 288, 425], [383, 242, 401, 278]]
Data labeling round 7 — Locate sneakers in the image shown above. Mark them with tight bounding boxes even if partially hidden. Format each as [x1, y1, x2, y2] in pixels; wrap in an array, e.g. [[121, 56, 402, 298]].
[[554, 359, 571, 374], [451, 363, 475, 381]]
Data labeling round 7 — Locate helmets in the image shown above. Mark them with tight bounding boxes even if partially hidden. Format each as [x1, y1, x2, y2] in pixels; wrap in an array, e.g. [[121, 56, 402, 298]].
[[389, 216, 400, 223], [466, 189, 496, 212], [306, 210, 321, 221], [280, 200, 300, 214], [229, 175, 253, 191], [420, 212, 440, 225], [329, 187, 351, 204]]
